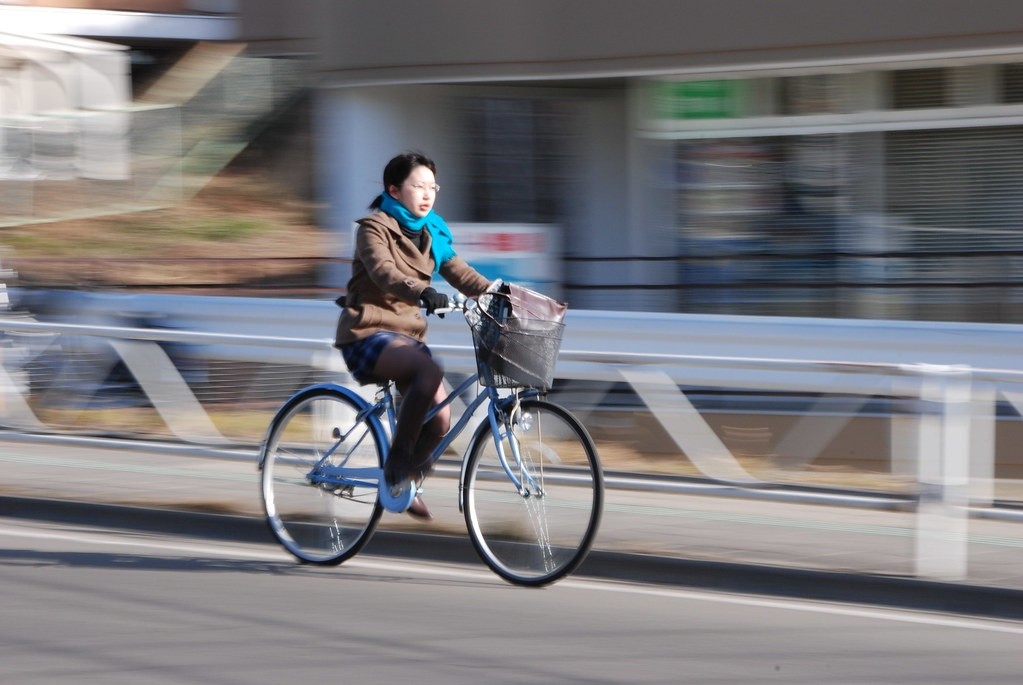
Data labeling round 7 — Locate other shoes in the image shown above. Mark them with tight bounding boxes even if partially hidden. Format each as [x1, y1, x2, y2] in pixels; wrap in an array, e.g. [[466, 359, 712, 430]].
[[406, 495, 434, 523]]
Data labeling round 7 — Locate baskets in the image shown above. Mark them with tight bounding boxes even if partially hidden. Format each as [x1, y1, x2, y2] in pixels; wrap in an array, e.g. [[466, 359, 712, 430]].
[[470, 317, 566, 390]]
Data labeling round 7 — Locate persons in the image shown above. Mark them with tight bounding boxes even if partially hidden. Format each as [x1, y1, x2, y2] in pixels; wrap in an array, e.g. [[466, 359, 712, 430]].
[[334, 153, 492, 523]]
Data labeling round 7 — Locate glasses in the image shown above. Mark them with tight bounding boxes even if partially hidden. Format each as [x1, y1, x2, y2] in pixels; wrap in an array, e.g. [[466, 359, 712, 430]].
[[405, 183, 442, 193]]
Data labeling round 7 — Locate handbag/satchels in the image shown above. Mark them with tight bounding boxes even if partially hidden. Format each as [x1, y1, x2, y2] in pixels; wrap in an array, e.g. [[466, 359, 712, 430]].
[[464, 282, 568, 392]]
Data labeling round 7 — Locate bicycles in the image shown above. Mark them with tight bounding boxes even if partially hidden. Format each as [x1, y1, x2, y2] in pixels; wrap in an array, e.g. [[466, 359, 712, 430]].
[[257, 294, 605, 588]]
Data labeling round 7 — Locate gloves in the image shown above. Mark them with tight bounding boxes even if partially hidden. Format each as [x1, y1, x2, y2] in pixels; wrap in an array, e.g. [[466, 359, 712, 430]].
[[420, 287, 449, 319]]
